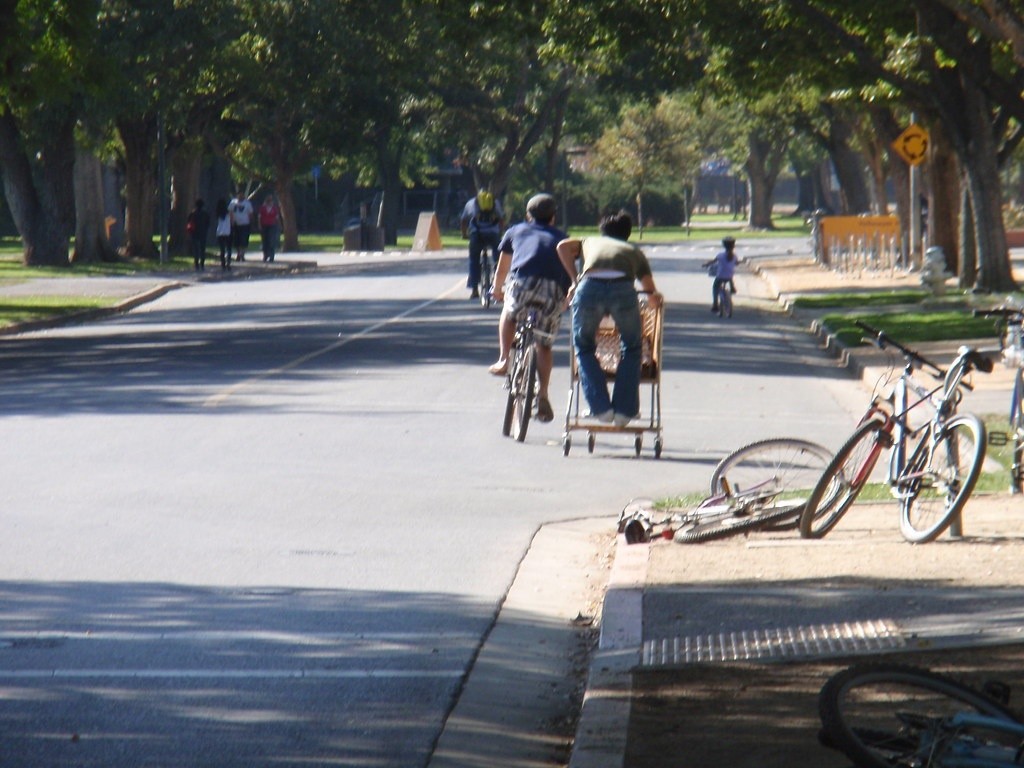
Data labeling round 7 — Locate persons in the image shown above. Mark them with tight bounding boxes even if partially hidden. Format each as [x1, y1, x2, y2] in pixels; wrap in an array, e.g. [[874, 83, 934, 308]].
[[489, 193, 571, 422], [701, 238, 738, 313], [187, 198, 210, 272], [216, 200, 233, 271], [227, 192, 253, 262], [557, 213, 662, 428], [258, 194, 280, 262], [460, 187, 504, 299]]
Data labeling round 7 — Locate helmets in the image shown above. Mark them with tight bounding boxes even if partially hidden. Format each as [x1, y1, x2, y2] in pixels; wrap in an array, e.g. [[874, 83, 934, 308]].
[[723, 236, 735, 242]]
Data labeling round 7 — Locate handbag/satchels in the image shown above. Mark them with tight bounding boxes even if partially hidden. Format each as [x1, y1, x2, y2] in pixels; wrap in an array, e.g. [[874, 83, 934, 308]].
[[186, 221, 195, 233]]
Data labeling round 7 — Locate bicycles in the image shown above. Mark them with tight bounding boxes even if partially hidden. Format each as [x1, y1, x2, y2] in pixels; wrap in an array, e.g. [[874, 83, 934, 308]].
[[488, 286, 549, 442], [613, 437, 844, 544], [463, 235, 494, 311], [814, 655, 1024, 768], [705, 265, 736, 319], [972, 303, 1023, 493], [799, 318, 996, 545]]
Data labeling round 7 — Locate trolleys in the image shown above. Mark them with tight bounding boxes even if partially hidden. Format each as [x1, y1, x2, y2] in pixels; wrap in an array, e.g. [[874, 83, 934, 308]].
[[558, 285, 667, 460]]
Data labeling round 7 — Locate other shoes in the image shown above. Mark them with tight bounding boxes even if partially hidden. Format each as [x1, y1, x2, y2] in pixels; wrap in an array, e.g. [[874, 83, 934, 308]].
[[489, 361, 508, 374], [201, 264, 204, 270], [227, 265, 231, 271], [536, 394, 554, 423], [221, 265, 225, 271], [242, 256, 246, 262], [581, 407, 613, 424], [471, 292, 479, 300], [614, 412, 640, 426], [270, 256, 274, 263], [710, 306, 720, 313], [236, 255, 242, 262], [194, 262, 199, 270], [263, 256, 267, 262]]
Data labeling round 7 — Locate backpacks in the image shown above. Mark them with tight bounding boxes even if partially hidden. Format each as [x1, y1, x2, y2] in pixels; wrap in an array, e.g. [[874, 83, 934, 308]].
[[475, 190, 496, 222]]
